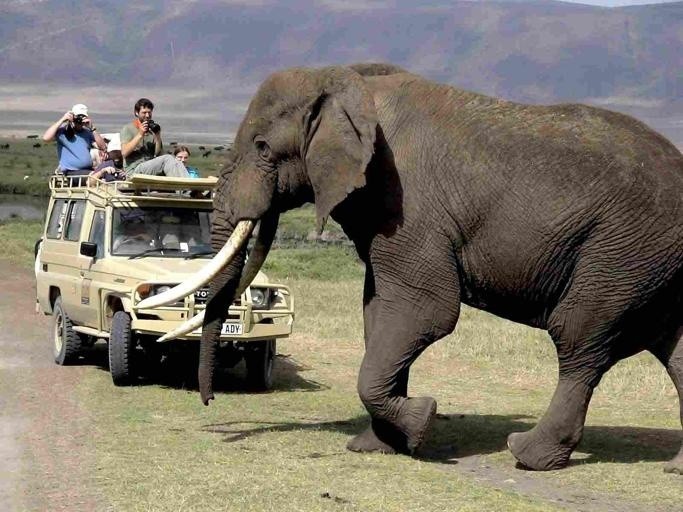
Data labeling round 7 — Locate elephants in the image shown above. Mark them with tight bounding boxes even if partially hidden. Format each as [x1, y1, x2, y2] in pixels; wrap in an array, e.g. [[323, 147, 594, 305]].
[[132, 63, 683, 475]]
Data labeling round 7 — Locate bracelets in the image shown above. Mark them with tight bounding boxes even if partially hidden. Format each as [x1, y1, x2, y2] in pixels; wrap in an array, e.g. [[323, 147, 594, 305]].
[[90, 128, 96, 132]]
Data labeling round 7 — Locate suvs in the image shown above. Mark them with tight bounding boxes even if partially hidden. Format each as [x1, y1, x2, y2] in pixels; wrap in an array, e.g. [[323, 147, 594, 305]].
[[32, 170, 295, 391]]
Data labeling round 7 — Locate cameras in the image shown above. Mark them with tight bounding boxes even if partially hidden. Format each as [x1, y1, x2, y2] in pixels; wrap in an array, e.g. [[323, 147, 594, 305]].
[[146, 120, 161, 134], [112, 171, 120, 178], [73, 115, 83, 125]]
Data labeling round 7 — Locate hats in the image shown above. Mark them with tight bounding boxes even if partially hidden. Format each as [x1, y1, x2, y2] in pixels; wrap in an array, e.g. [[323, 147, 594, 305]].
[[73, 103, 88, 118]]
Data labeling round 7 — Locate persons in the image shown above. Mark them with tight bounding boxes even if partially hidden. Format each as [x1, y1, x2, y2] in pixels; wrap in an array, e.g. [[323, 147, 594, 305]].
[[41, 103, 107, 187], [112, 216, 153, 255], [176, 213, 209, 246], [81, 141, 125, 187], [119, 97, 211, 198], [171, 144, 200, 178]]
